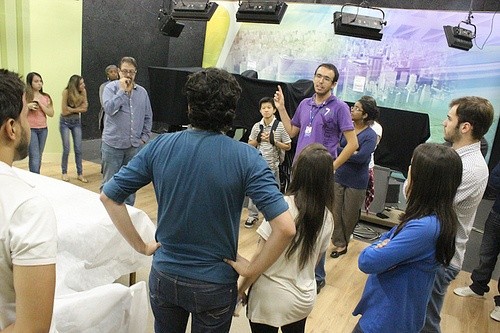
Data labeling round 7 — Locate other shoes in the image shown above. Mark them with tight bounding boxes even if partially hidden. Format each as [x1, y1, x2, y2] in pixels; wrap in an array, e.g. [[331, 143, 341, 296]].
[[62, 174, 70, 182], [489, 306, 500, 321], [330, 246, 348, 258], [245, 216, 256, 228], [78, 175, 88, 183], [453, 286, 484, 297]]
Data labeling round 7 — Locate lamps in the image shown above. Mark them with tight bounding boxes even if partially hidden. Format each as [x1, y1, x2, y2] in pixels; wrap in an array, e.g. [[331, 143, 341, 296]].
[[331, 0, 387, 41], [236, 0, 288, 24], [157, 8, 185, 39], [443, 0, 476, 51], [171, 0, 219, 22]]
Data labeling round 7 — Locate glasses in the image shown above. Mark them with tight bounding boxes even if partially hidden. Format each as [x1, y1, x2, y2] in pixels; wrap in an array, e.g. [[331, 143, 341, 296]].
[[351, 106, 364, 112], [120, 68, 136, 76]]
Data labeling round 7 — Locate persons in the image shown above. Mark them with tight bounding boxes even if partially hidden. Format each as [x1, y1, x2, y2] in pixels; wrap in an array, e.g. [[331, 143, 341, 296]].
[[349, 94, 382, 240], [351, 143, 463, 333], [403, 97, 493, 333], [59, 75, 88, 184], [100, 67, 296, 333], [454, 165, 500, 320], [102, 57, 152, 207], [0, 67, 56, 333], [20, 71, 54, 174], [329, 100, 378, 259], [273, 62, 359, 292], [234, 143, 334, 333], [244, 97, 291, 228], [97, 65, 119, 174]]
